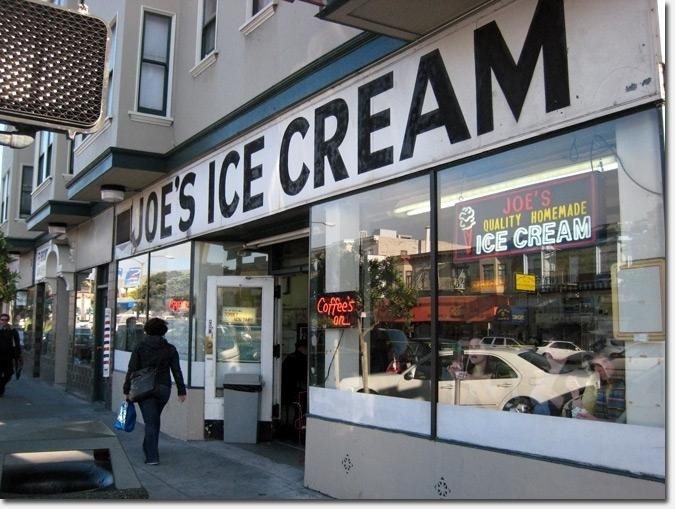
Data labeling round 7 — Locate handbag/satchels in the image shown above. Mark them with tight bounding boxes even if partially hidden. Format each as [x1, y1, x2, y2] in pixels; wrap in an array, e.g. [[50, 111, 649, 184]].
[[126, 365, 161, 404], [113, 399, 139, 432]]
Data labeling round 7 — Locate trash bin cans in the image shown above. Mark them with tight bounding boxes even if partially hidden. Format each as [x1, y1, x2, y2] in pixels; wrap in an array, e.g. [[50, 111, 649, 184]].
[[222, 373, 262, 445]]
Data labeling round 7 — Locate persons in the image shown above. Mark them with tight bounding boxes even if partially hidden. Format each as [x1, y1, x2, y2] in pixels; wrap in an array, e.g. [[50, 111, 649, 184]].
[[123, 318, 187, 466], [280, 338, 312, 420], [0, 314, 25, 399], [114, 316, 136, 352], [548, 339, 628, 421], [446, 336, 503, 382]]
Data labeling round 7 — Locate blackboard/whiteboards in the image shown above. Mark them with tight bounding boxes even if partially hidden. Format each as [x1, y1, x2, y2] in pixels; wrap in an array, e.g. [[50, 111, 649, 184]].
[[609, 257, 666, 341], [312, 291, 356, 330]]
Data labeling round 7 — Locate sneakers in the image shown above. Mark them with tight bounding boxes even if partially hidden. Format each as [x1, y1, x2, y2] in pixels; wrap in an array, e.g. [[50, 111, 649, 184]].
[[144, 457, 160, 465]]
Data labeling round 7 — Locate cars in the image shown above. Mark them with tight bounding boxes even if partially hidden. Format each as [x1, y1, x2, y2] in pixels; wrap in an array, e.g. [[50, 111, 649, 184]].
[[339, 334, 665, 383], [341, 343, 600, 415], [40, 314, 261, 388]]
